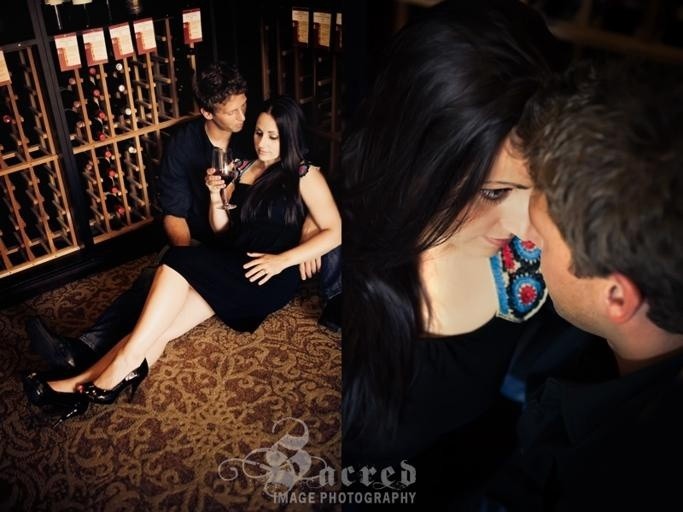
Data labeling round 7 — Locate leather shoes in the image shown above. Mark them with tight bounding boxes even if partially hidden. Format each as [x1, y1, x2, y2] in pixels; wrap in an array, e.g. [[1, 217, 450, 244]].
[[318, 293, 343, 334], [26, 316, 74, 375]]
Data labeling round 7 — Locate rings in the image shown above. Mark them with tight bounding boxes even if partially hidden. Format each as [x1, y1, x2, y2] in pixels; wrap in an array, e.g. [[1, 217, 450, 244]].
[[263, 268, 267, 274]]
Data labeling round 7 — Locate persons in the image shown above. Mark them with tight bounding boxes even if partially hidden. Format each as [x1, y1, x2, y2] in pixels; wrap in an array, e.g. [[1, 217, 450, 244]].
[[344, 0, 560, 510], [18, 93, 342, 430], [15, 58, 341, 379], [467, 42, 683, 512]]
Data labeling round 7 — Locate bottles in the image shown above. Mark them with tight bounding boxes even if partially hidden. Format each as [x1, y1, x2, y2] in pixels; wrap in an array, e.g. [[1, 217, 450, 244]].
[[0, 21, 343, 282]]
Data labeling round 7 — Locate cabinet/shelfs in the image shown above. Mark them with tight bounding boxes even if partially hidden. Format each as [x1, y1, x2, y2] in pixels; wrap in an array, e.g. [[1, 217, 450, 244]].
[[234, 0, 366, 188], [0, 0, 97, 309], [36, 0, 234, 272]]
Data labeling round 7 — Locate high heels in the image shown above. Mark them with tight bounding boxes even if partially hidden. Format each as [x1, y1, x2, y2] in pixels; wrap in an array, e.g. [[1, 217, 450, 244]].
[[23, 371, 90, 430], [76, 358, 150, 405]]
